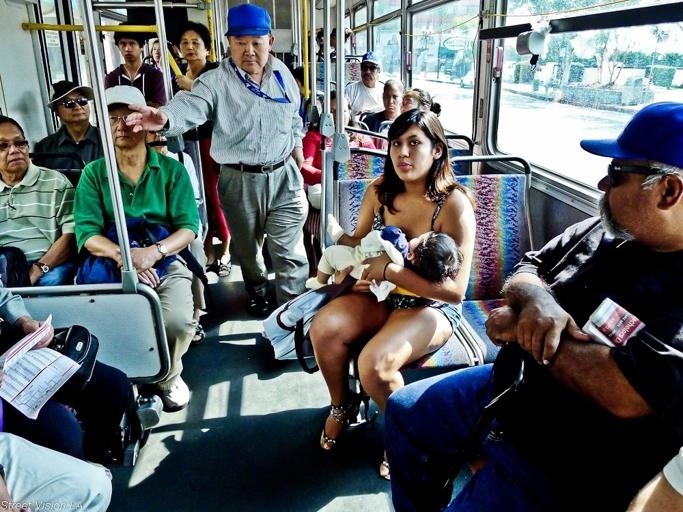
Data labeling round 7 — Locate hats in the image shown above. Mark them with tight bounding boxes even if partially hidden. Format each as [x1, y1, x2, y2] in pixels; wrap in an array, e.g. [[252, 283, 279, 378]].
[[47, 81, 94, 107], [362, 52, 380, 65], [225, 4, 271, 36], [580, 102, 683, 168], [106, 85, 146, 106]]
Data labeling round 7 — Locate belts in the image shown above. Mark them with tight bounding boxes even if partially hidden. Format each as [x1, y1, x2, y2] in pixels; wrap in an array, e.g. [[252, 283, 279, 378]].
[[224, 155, 290, 173]]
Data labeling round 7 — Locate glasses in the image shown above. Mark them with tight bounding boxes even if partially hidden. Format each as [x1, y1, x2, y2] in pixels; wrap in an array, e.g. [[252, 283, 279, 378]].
[[0, 141, 28, 150], [608, 165, 679, 188], [59, 96, 88, 108], [110, 115, 127, 125]]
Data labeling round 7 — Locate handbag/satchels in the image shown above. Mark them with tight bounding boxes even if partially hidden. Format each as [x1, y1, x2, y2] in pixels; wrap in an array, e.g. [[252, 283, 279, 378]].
[[262, 284, 350, 373], [48, 324, 99, 393], [77, 217, 175, 285]]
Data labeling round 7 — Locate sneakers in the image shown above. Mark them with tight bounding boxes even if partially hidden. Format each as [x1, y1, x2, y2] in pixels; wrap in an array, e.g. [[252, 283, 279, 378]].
[[248, 296, 270, 317], [164, 376, 189, 410]]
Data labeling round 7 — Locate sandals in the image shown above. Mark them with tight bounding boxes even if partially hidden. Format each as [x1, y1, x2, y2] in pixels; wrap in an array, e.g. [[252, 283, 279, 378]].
[[192, 324, 205, 345], [320, 392, 360, 450], [219, 258, 231, 277]]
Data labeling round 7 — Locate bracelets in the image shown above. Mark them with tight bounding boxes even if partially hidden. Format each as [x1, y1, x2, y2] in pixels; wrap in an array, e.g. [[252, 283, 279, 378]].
[[382, 259, 394, 280]]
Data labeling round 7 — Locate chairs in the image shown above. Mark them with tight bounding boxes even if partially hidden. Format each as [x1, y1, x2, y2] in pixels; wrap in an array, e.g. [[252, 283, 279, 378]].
[[292, 54, 540, 439], [162, 128, 205, 244]]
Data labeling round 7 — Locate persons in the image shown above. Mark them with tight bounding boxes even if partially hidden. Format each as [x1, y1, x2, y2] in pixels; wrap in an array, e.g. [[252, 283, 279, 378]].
[[387, 99, 683, 510], [0, 431, 113, 510], [305, 108, 477, 480], [307, 228, 459, 296], [0, 4, 441, 456], [626, 442, 682, 509]]
